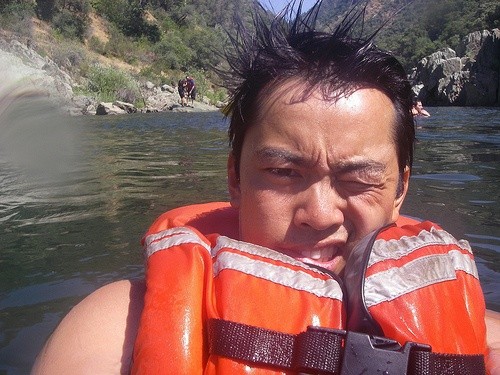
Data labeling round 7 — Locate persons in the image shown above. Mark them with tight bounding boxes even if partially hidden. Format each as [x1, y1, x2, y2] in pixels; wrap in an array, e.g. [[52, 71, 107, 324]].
[[26, 1, 499, 374], [411, 98, 431, 119], [178, 78, 187, 107], [185, 76, 196, 108]]
